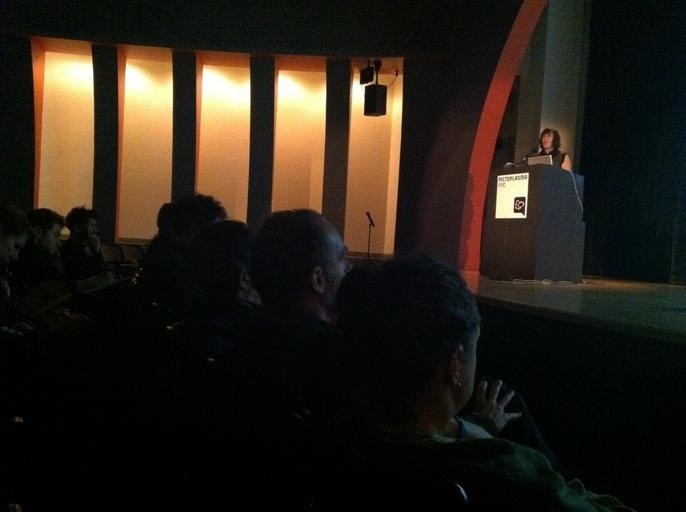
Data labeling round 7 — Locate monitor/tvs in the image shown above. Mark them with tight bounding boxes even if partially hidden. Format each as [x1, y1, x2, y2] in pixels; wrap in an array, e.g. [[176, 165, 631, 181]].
[[527, 155, 553, 166]]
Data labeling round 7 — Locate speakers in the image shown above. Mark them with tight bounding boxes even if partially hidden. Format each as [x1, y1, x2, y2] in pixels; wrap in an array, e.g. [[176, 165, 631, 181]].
[[363, 84, 388, 116]]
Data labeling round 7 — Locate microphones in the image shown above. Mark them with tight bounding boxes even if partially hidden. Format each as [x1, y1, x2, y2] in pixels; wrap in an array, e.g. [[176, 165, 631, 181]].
[[366, 211, 376, 227], [521, 147, 539, 161]]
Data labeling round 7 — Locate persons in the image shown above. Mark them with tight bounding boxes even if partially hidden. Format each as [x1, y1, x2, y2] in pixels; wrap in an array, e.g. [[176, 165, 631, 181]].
[[1, 194, 635, 512], [504, 128, 571, 170]]
